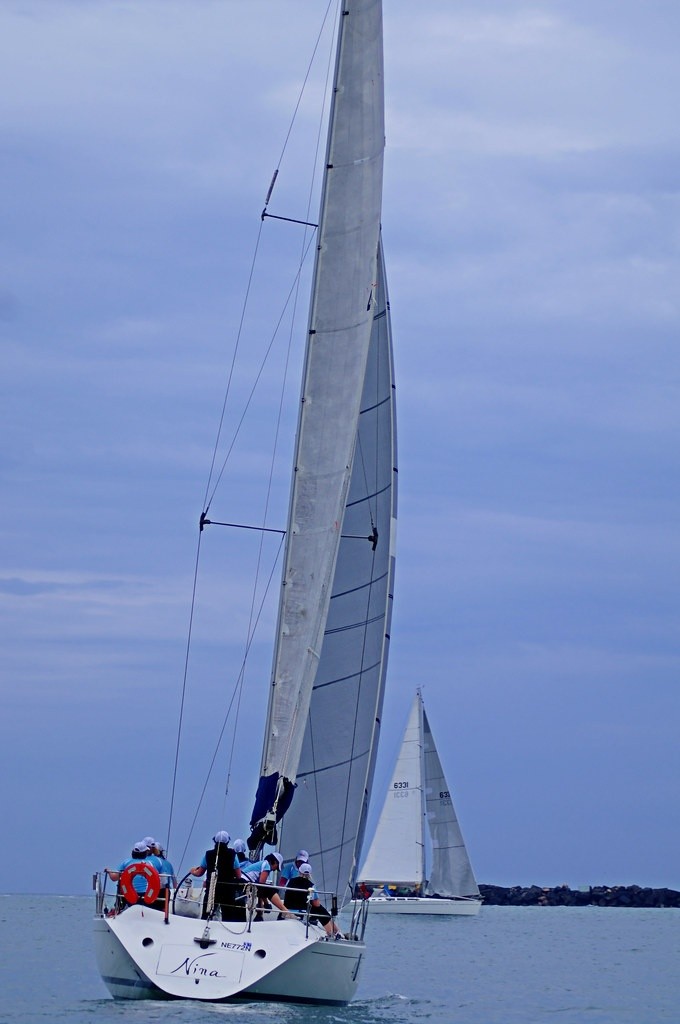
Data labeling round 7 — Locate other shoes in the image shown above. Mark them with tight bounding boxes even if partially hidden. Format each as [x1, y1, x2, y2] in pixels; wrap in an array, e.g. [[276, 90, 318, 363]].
[[336, 931, 346, 939]]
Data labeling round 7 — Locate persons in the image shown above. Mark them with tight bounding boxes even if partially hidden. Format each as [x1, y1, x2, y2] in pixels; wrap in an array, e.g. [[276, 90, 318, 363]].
[[283, 863, 345, 940], [230, 851, 301, 923], [353, 881, 371, 900], [188, 830, 242, 923], [152, 842, 177, 906], [277, 849, 309, 921], [103, 841, 160, 909], [232, 838, 256, 874], [141, 836, 170, 911]]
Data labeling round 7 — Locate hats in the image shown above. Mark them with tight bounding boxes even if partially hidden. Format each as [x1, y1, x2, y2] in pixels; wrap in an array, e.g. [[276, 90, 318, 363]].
[[212, 831, 246, 853], [296, 850, 309, 862], [299, 864, 312, 874], [133, 837, 167, 854], [272, 852, 282, 871]]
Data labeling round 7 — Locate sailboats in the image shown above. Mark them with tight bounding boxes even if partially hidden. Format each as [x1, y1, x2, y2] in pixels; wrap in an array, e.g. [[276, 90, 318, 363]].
[[360, 694, 483, 921], [102, 0, 403, 1012]]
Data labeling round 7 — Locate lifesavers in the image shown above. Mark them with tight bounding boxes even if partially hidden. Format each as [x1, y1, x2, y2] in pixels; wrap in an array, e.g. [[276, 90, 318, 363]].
[[119, 862, 161, 903]]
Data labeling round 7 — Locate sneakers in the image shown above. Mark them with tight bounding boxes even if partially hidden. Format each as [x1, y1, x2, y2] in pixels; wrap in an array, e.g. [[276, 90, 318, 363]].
[[281, 913, 300, 920]]
